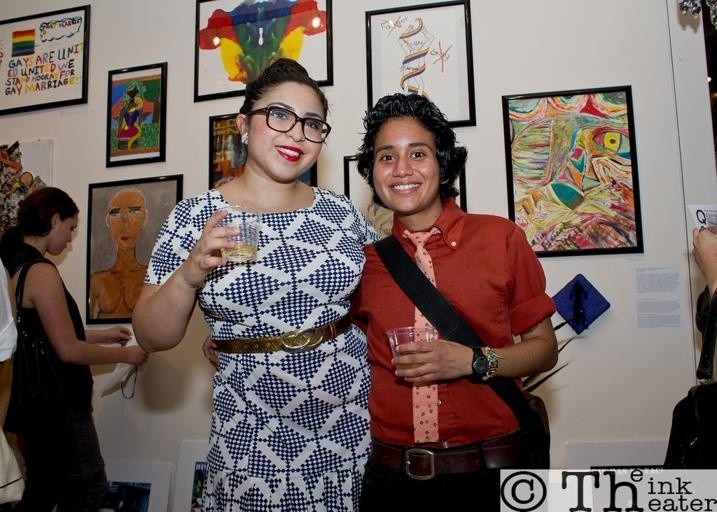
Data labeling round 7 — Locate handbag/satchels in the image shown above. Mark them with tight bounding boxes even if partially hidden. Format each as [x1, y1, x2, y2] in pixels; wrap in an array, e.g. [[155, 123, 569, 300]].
[[522, 393, 550, 468], [665, 381, 715, 470]]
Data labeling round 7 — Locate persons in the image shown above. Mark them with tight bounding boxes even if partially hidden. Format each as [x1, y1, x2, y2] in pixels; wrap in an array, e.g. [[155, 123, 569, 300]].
[[0, 253, 20, 434], [89, 187, 152, 319], [3, 186, 150, 512], [129, 57, 386, 512], [198, 91, 559, 511], [664, 225, 717, 470]]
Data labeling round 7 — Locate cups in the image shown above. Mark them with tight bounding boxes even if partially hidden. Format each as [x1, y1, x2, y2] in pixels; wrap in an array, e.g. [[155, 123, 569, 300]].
[[385, 325, 435, 374], [215, 203, 263, 264]]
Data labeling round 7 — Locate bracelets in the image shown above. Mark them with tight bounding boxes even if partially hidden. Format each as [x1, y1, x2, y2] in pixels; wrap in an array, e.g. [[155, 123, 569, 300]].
[[484, 347, 503, 380]]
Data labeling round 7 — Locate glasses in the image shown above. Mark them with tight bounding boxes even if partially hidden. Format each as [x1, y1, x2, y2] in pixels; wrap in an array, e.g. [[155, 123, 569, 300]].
[[243, 106, 331, 143]]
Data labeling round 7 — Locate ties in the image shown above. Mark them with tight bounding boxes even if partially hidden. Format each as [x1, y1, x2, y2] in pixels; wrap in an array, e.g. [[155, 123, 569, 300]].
[[402, 227, 442, 443]]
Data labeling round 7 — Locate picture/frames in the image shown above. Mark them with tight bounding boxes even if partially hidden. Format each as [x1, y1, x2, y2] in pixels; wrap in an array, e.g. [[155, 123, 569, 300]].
[[359, 0, 476, 127], [563, 437, 672, 512], [500, 84, 644, 257], [97, 459, 173, 512], [1, 4, 91, 115], [342, 149, 467, 234], [208, 112, 316, 215], [194, 0, 333, 99], [172, 437, 214, 512], [105, 61, 168, 169], [88, 172, 184, 323]]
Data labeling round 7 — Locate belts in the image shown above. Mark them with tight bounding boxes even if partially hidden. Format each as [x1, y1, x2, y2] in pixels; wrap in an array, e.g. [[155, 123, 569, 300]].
[[370, 441, 518, 481], [213, 313, 351, 353]]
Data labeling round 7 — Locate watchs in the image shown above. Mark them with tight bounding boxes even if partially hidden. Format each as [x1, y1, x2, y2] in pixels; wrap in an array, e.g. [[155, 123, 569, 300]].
[[470, 344, 487, 377]]
